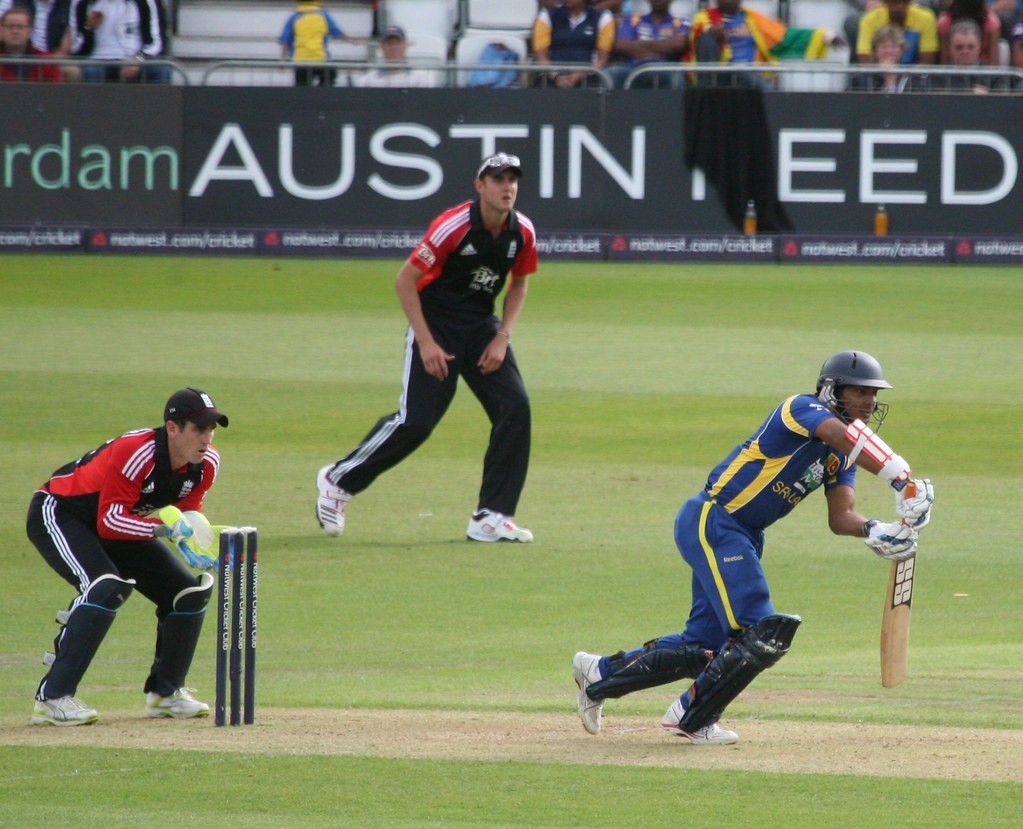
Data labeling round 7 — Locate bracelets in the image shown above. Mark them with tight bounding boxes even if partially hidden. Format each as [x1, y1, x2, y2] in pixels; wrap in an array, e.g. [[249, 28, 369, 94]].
[[498, 331, 511, 344]]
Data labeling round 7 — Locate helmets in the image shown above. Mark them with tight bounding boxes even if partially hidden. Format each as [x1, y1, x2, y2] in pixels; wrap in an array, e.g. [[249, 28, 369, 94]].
[[817, 351, 893, 431]]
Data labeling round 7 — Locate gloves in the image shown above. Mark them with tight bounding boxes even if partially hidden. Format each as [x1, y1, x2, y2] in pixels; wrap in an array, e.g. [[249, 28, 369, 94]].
[[865, 522, 917, 561], [896, 476, 934, 529]]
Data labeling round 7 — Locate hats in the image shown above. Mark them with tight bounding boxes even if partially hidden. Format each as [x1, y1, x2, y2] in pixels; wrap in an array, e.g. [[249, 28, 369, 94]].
[[477, 153, 522, 179], [164, 387, 228, 427], [383, 25, 402, 41]]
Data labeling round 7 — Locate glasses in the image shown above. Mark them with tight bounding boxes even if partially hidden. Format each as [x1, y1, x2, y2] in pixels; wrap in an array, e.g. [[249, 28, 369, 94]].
[[478, 158, 520, 177]]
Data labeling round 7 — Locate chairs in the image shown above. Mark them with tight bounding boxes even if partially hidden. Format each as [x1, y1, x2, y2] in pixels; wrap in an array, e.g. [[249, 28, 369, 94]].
[[370, 0, 856, 93]]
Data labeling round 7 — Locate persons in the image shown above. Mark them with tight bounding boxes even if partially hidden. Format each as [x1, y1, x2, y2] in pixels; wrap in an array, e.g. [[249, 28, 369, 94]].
[[278, 0, 365, 87], [842, 0, 1023, 93], [26, 386, 229, 727], [357, 26, 435, 88], [0, 0, 173, 84], [573, 351, 934, 745], [315, 151, 537, 543], [532, 0, 846, 93]]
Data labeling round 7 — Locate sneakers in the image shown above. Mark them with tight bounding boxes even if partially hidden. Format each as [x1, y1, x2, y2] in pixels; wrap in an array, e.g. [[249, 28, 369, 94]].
[[316, 464, 351, 536], [467, 508, 533, 543], [661, 701, 739, 745], [146, 687, 209, 719], [33, 694, 98, 726], [573, 651, 606, 735]]
